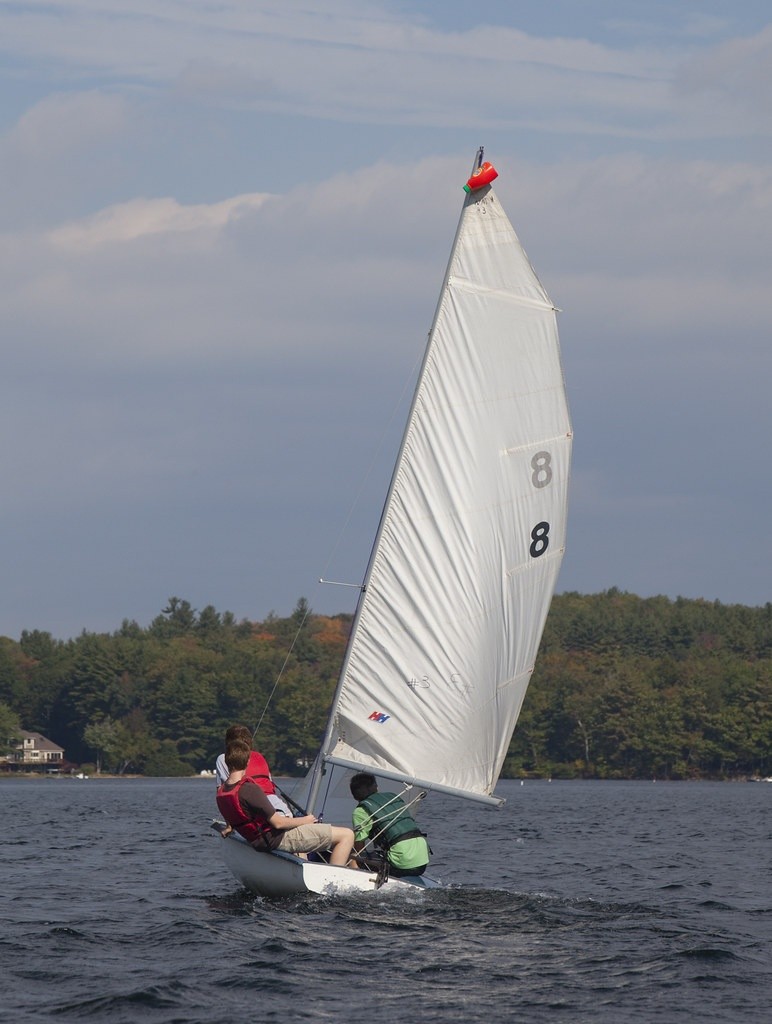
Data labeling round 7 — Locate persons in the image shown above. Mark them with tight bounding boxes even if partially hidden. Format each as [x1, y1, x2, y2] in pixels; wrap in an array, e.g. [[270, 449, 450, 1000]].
[[350, 772, 429, 876], [216, 738, 355, 868], [216, 725, 308, 862]]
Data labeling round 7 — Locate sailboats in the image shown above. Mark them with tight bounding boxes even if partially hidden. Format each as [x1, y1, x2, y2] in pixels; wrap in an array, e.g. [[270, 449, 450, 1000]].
[[210, 144, 573, 905]]
